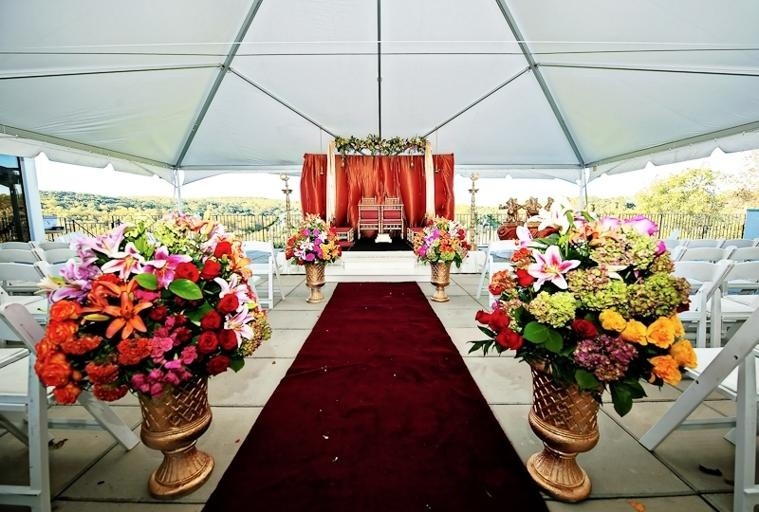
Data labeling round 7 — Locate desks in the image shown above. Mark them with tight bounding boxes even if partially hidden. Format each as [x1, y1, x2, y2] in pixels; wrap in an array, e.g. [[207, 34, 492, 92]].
[[357, 195, 405, 240]]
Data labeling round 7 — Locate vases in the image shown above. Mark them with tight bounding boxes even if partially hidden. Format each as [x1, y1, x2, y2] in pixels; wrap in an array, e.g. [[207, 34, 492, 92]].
[[305, 262, 327, 304], [430, 261, 451, 303]]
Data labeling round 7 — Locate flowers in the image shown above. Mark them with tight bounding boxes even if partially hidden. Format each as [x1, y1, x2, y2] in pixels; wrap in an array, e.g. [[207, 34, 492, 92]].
[[413, 216, 473, 269], [34, 210, 273, 411], [468, 198, 700, 418], [286, 214, 343, 265]]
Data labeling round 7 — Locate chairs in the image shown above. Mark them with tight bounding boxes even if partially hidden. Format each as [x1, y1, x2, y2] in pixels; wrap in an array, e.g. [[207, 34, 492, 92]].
[[326, 215, 354, 243], [475, 236, 759, 511], [407, 214, 436, 243], [1, 232, 285, 512]]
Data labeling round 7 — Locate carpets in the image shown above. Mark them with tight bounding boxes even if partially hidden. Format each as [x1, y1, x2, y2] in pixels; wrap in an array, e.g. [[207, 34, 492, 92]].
[[202, 281, 551, 511]]
